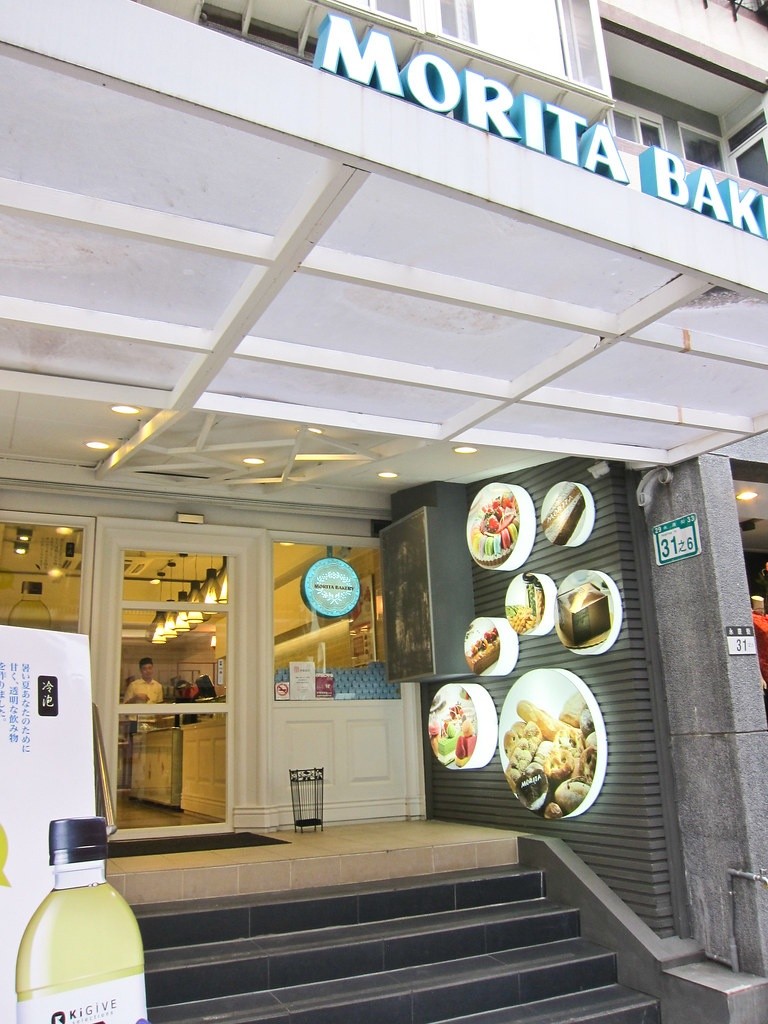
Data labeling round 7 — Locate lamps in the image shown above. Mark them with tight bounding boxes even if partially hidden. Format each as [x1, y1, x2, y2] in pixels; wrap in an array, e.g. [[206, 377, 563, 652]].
[[144, 553, 228, 644], [16, 525, 34, 541], [14, 542, 29, 555]]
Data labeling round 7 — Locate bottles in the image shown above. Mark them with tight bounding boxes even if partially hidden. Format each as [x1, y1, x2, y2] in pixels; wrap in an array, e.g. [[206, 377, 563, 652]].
[[15, 817, 148, 1024]]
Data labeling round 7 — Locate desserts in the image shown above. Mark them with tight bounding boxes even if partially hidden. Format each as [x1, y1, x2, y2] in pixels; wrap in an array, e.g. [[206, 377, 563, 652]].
[[428, 687, 477, 767], [463, 628, 500, 675]]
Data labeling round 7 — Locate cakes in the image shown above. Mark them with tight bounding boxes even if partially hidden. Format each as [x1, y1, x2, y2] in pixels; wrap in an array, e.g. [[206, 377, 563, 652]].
[[470, 492, 520, 566]]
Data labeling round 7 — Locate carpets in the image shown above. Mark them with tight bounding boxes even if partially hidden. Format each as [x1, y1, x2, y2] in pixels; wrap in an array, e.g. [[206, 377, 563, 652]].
[[106, 830, 292, 858]]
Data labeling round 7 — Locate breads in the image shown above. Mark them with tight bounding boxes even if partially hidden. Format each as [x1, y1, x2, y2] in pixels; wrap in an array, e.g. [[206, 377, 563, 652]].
[[503, 693, 596, 819]]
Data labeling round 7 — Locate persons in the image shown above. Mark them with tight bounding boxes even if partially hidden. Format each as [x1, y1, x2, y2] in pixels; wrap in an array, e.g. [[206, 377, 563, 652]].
[[124, 658, 163, 735], [752, 596, 768, 716]]
